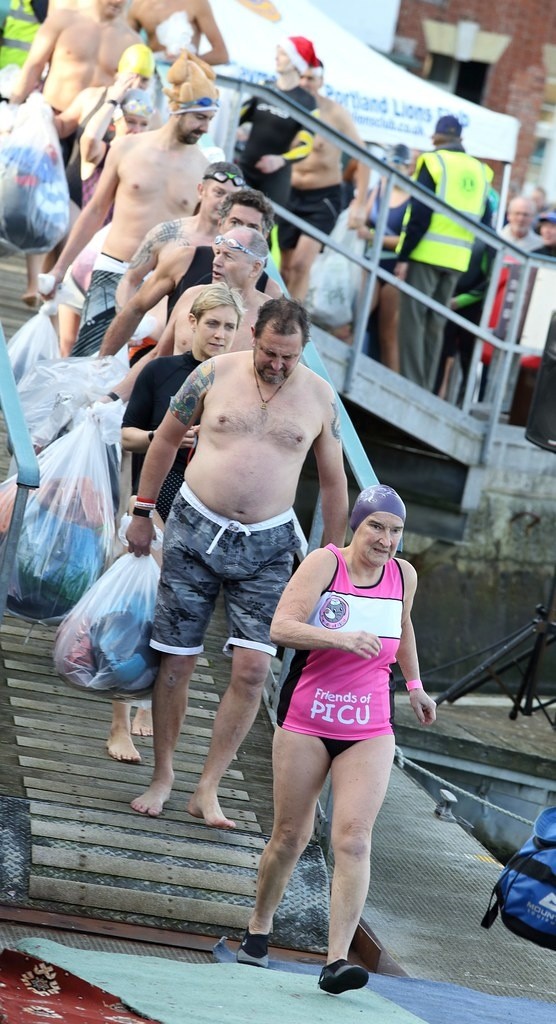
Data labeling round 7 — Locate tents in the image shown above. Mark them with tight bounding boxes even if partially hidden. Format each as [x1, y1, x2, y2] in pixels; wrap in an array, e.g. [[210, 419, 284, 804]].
[[199, 0, 522, 231]]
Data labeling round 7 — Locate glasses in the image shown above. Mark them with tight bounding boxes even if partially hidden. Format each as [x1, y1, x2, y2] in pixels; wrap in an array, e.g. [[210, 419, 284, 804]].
[[122, 99, 155, 116], [381, 157, 402, 164], [202, 171, 244, 187], [177, 98, 219, 107], [213, 235, 265, 266]]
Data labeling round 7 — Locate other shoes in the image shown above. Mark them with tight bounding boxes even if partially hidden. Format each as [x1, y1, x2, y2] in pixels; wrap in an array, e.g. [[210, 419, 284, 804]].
[[319, 959, 369, 995], [237, 926, 268, 967]]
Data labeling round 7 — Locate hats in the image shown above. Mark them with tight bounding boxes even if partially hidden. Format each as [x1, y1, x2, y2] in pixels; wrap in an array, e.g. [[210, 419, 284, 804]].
[[119, 89, 154, 117], [162, 48, 220, 114], [349, 484, 407, 531], [532, 208, 555, 234], [278, 37, 323, 76], [118, 44, 155, 79], [435, 115, 462, 137]]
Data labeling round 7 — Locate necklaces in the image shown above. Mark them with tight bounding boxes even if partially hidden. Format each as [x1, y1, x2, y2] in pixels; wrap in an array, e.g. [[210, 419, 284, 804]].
[[253, 366, 287, 411]]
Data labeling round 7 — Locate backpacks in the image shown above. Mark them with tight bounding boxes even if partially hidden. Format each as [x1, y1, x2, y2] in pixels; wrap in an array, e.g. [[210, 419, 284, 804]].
[[480, 807, 556, 951]]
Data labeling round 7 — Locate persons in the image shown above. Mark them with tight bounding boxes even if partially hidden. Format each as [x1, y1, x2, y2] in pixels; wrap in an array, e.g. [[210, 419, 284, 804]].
[[236, 34, 556, 454], [125, 294, 349, 830], [0, 0, 229, 358], [103, 282, 243, 762], [114, 161, 248, 316], [93, 188, 285, 366], [89, 227, 278, 408], [236, 486, 436, 995]]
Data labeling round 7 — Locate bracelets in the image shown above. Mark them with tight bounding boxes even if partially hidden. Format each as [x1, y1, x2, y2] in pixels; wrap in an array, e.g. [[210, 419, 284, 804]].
[[100, 97, 118, 109], [405, 679, 424, 692], [106, 391, 120, 401], [148, 429, 153, 443], [132, 496, 157, 521]]
